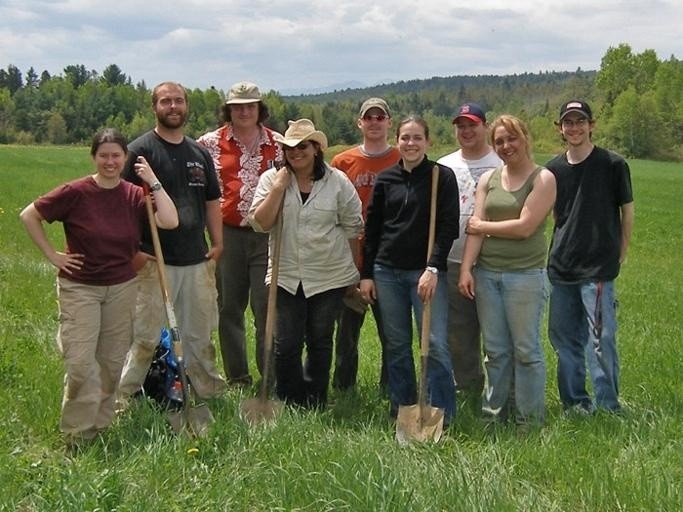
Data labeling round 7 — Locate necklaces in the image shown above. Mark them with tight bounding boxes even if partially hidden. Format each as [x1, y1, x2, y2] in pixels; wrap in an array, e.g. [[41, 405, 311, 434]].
[[361, 146, 391, 157]]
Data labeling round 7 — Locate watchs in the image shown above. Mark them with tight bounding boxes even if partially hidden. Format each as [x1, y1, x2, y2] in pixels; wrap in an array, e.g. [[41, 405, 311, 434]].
[[426, 267, 440, 275], [151, 182, 162, 192]]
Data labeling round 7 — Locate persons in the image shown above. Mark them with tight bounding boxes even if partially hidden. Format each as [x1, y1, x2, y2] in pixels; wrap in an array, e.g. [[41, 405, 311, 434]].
[[457, 115, 558, 444], [360, 115, 460, 432], [434, 102, 506, 402], [195, 82, 289, 393], [329, 97, 402, 406], [19, 129, 179, 458], [121, 80, 231, 414], [248, 117, 365, 417], [544, 102, 634, 426]]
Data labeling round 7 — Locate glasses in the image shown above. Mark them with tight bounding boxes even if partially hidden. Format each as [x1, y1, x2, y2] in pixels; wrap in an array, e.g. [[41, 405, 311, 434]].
[[282, 142, 312, 151], [564, 120, 587, 126], [364, 114, 389, 122]]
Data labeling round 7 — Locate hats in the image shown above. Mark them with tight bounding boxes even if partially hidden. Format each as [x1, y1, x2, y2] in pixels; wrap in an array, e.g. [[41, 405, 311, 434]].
[[360, 98, 390, 120], [560, 101, 591, 121], [272, 119, 328, 153], [226, 82, 261, 105], [453, 102, 485, 124]]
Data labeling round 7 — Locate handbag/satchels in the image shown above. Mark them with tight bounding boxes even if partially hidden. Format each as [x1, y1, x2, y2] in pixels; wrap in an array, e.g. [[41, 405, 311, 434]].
[[141, 327, 190, 413]]
